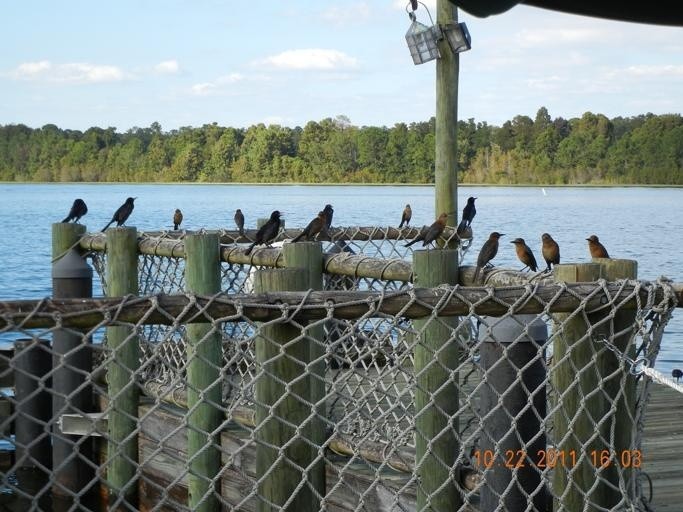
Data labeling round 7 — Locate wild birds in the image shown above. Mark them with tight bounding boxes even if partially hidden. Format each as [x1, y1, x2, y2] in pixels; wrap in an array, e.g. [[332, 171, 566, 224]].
[[585, 235, 610, 258], [174, 209, 183, 230], [471, 232, 506, 284], [399, 197, 478, 249], [61, 199, 87, 225], [542, 233, 560, 270], [510, 238, 539, 273], [234, 204, 334, 256], [671, 368, 682, 385], [100, 196, 138, 232]]
